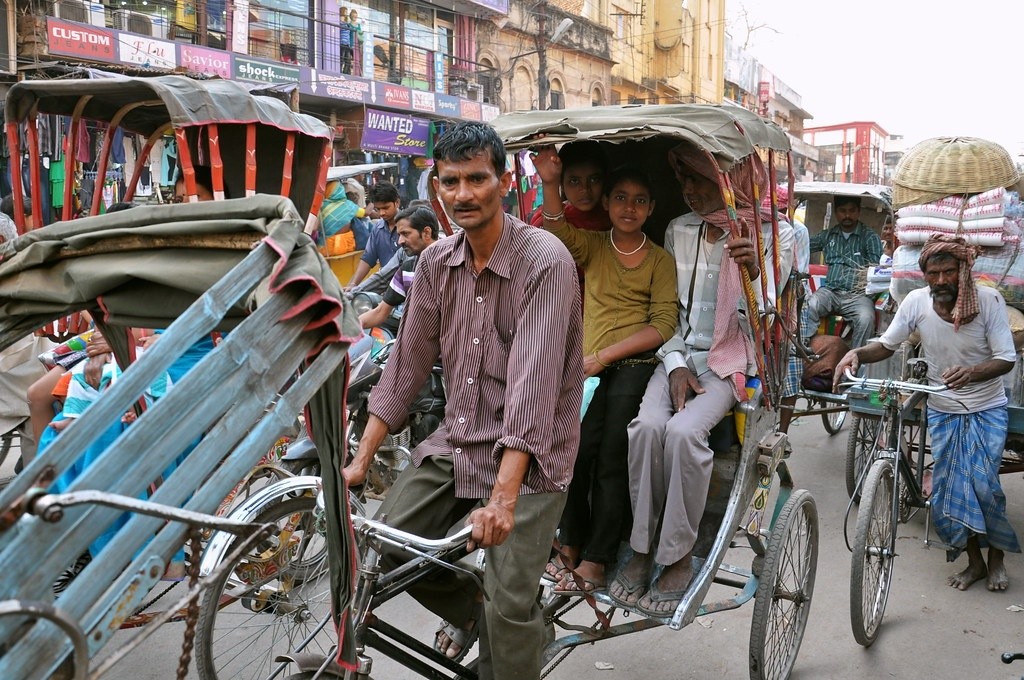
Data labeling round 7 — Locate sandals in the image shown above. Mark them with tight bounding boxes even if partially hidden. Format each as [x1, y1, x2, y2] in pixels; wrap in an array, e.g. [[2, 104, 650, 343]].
[[433, 600, 485, 663]]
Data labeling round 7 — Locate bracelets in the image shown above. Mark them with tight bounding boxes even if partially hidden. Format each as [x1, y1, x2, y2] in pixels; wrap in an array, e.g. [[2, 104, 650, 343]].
[[543, 208, 564, 217], [593, 349, 612, 368], [541, 211, 566, 221]]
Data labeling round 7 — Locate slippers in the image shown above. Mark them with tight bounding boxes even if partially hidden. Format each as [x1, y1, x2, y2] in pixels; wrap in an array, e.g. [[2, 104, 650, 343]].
[[608, 562, 657, 609], [552, 571, 608, 596], [637, 572, 694, 617], [542, 553, 575, 582]]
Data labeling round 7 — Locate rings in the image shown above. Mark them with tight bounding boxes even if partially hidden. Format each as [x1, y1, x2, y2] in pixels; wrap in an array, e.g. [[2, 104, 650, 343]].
[[91, 377, 97, 380]]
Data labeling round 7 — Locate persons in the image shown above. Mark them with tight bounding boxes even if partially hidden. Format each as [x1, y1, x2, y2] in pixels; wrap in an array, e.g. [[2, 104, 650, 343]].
[[831, 231, 1024, 591], [339, 6, 365, 76], [0, 165, 250, 615], [317, 119, 900, 680]]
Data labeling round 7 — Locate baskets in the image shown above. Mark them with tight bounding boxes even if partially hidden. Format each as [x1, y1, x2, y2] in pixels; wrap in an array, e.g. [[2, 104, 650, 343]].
[[891, 136, 1019, 210]]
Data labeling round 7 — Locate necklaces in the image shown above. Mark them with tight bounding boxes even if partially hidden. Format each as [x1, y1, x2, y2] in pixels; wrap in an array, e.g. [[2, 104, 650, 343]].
[[609, 226, 646, 256]]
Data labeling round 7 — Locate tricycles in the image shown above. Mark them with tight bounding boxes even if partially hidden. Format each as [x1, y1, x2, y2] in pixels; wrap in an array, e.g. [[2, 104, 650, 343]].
[[0, 75, 901, 680], [830, 337, 1024, 645]]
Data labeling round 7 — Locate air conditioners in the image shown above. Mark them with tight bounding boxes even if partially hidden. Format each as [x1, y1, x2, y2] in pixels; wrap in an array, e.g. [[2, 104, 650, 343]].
[[44, 0, 169, 41], [448, 79, 483, 104]]
